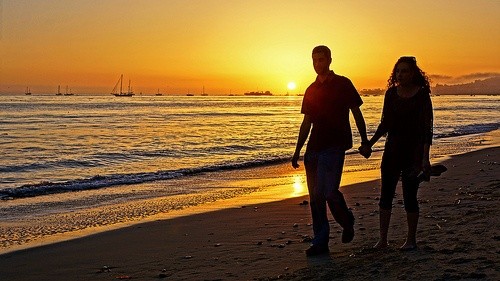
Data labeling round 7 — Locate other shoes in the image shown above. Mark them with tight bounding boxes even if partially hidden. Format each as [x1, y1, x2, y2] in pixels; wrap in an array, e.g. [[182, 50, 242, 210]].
[[399, 242, 416, 250], [306, 242, 329, 256], [342, 226, 355, 244], [373, 241, 390, 248]]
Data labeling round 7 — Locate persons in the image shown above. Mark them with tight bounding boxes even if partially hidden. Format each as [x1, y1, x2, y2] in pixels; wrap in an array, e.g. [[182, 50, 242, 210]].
[[292, 45, 371, 259], [358, 57, 434, 251]]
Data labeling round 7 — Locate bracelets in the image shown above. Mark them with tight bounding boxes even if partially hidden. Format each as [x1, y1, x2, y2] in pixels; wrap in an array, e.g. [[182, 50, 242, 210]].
[[361, 140, 369, 145], [295, 149, 300, 152]]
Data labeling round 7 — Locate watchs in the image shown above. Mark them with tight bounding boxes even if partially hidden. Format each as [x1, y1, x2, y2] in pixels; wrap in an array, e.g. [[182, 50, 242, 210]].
[[422, 162, 430, 167]]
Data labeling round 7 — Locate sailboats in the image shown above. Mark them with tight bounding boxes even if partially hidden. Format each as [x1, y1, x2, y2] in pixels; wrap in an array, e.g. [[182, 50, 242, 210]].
[[24, 83, 275, 96], [111, 74, 136, 96]]
